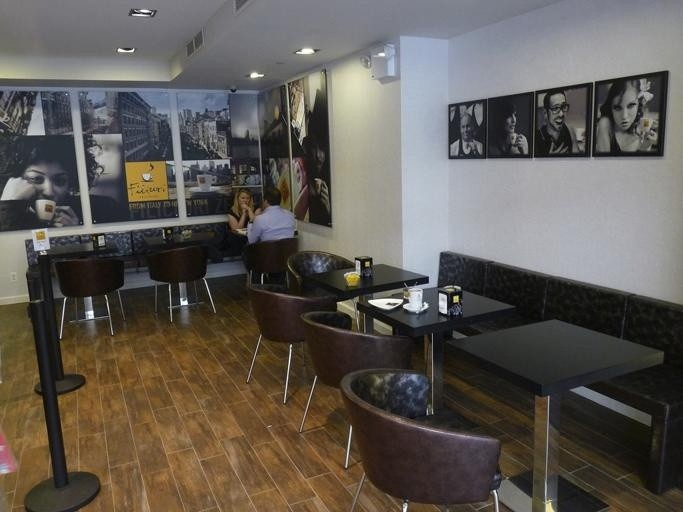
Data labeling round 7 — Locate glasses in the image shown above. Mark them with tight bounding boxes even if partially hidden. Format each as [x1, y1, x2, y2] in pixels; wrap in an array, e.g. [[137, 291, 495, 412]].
[[546, 103, 570, 114]]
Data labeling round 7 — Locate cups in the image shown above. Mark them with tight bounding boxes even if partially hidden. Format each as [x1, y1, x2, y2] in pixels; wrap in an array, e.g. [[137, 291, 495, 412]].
[[142, 174, 153, 182], [409, 288, 424, 309], [196, 174, 217, 192], [638, 117, 654, 133], [28, 199, 57, 220], [314, 178, 322, 194], [506, 134, 517, 146], [573, 127, 586, 143], [343, 272, 360, 287], [403, 287, 409, 300]]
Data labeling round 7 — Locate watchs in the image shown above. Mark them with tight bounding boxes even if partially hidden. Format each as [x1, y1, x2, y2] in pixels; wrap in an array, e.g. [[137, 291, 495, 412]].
[[248, 218, 253, 223]]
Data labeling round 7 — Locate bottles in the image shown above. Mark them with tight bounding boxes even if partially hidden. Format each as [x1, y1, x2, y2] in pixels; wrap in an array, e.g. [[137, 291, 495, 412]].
[[250, 162, 258, 174], [231, 164, 237, 180], [239, 163, 249, 175]]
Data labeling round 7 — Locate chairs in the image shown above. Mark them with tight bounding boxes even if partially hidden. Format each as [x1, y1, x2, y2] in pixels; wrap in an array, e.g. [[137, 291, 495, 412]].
[[56, 260, 127, 339], [241, 238, 299, 291], [298, 311, 411, 468], [283, 250, 355, 294], [340, 369, 504, 507], [453, 317, 666, 508], [148, 246, 218, 323], [244, 283, 336, 403]]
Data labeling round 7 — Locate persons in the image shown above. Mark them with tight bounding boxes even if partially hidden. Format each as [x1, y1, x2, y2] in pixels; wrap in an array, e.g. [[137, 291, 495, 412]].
[[487, 97, 529, 155], [288, 147, 332, 227], [449, 109, 484, 157], [535, 89, 586, 154], [594, 76, 659, 152], [0, 133, 105, 233], [246, 184, 300, 285], [242, 174, 252, 185], [228, 187, 256, 273]]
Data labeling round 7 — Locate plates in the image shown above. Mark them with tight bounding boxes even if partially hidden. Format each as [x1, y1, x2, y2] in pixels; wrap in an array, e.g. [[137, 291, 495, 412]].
[[367, 298, 404, 312], [404, 302, 427, 315], [189, 187, 220, 193]]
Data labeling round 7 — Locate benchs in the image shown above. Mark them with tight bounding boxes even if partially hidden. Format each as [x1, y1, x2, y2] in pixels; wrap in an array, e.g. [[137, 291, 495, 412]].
[[436, 251, 682, 495], [25, 221, 232, 301]]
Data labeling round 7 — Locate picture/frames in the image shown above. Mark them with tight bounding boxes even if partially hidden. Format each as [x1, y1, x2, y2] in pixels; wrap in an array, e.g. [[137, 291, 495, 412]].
[[533, 84, 590, 158], [447, 100, 485, 160], [488, 92, 533, 159], [591, 70, 667, 156]]
